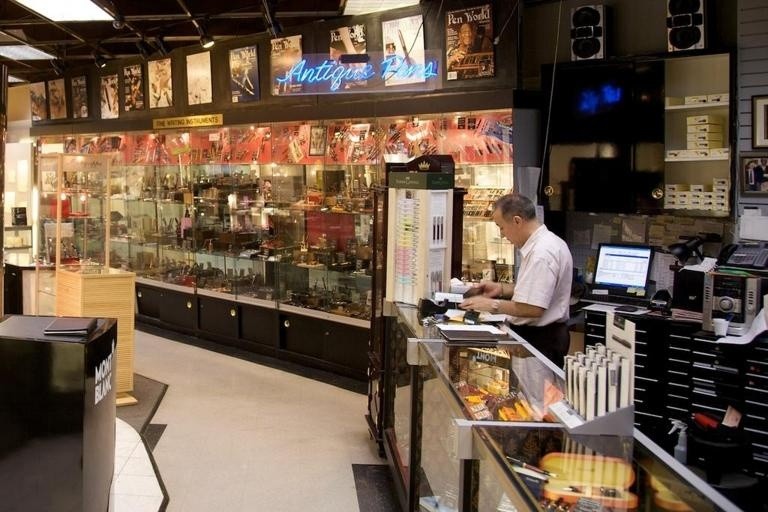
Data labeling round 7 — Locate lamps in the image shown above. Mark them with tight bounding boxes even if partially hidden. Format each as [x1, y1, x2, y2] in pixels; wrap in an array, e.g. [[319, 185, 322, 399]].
[[0, 0, 279, 78]]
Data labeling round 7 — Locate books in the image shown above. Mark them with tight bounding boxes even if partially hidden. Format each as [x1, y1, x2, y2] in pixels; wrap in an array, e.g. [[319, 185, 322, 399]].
[[43, 317, 97, 338], [435, 324, 507, 342]]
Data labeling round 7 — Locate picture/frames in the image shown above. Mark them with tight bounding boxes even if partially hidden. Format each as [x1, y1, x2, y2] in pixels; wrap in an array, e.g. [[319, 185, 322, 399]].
[[751, 94, 768, 149], [739, 152, 768, 196]]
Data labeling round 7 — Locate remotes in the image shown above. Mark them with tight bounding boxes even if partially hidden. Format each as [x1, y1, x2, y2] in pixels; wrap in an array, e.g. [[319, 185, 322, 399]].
[[754, 249, 768, 267]]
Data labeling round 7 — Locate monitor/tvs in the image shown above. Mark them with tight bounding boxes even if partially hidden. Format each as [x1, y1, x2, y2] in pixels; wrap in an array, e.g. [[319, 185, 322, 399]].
[[541, 59, 665, 143], [592, 243, 654, 288]]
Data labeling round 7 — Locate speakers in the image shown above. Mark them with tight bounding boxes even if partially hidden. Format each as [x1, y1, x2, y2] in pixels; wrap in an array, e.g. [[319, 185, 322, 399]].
[[568, 4, 606, 62], [666, 0, 705, 53]]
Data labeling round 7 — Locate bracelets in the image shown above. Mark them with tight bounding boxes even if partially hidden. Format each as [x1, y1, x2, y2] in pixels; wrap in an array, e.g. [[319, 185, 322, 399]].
[[488, 299, 500, 315], [500, 283, 503, 298]]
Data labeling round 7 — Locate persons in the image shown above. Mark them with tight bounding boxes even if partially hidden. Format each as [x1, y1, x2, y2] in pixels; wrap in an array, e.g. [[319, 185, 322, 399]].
[[457, 194, 574, 369], [745, 158, 768, 191], [446, 23, 494, 72]]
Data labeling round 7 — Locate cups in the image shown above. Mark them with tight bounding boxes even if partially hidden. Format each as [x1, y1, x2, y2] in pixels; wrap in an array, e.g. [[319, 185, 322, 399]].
[[713, 319, 729, 336]]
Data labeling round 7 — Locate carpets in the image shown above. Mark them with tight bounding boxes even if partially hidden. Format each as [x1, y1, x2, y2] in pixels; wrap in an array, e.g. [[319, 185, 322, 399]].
[[143, 424, 167, 451], [402, 466, 438, 507], [352, 464, 403, 512]]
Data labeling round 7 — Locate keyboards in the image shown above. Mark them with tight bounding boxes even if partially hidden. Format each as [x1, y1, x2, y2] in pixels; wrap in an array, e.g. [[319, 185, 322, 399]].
[[580, 294, 649, 308]]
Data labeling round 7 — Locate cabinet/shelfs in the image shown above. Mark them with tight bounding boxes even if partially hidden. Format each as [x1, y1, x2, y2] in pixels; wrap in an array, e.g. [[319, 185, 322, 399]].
[[582, 303, 768, 482], [541, 48, 735, 218], [28, 88, 537, 381]]
